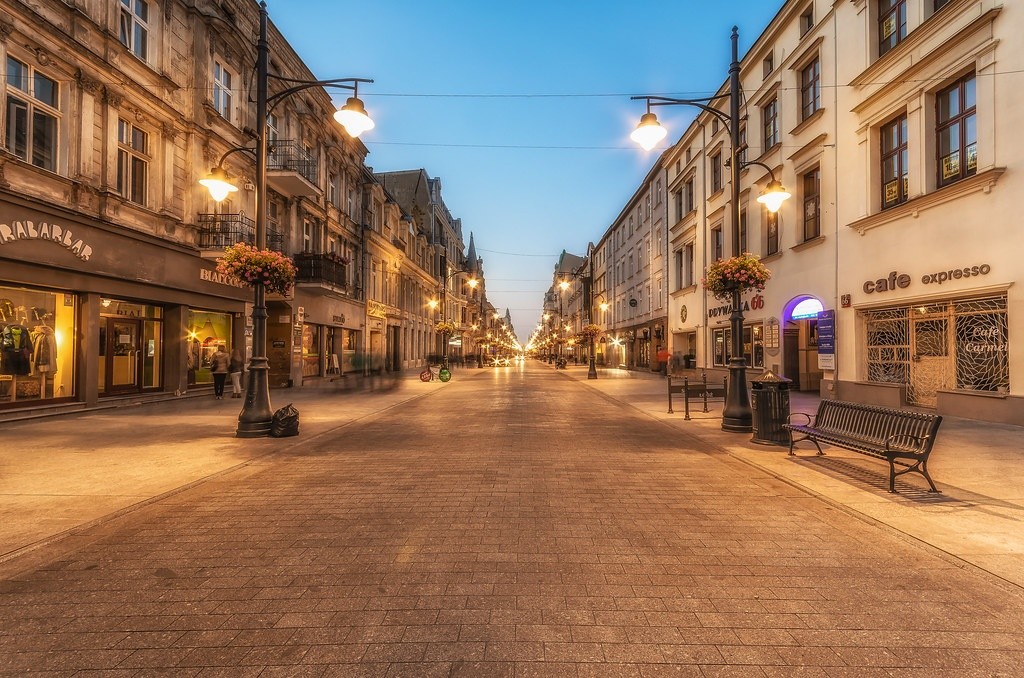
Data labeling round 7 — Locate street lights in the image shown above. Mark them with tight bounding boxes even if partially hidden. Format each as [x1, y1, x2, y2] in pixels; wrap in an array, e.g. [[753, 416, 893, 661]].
[[544, 310, 571, 357], [630, 24, 792, 432], [199, 0, 377, 439], [560, 250, 610, 379], [472, 308, 499, 368], [431, 246, 478, 373]]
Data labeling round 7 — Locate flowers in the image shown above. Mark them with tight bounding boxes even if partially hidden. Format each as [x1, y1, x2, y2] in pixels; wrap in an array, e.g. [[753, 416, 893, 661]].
[[434, 322, 454, 336], [584, 323, 602, 338], [216, 242, 296, 294], [701, 252, 771, 303]]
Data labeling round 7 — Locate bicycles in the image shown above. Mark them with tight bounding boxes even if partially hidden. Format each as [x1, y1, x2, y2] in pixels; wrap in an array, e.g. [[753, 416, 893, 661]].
[[420, 361, 451, 382]]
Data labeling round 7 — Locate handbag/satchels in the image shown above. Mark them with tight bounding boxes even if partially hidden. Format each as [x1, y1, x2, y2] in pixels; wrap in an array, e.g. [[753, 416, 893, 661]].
[[210, 353, 218, 372]]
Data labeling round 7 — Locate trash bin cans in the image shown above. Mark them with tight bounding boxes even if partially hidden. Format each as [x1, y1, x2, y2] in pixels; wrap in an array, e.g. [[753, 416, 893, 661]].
[[748, 370, 796, 447]]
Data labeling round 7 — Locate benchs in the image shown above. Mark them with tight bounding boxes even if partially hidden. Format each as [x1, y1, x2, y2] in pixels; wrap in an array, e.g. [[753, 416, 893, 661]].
[[783, 399, 945, 495]]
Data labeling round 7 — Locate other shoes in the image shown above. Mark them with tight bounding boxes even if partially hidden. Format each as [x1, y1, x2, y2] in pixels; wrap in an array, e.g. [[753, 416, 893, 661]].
[[219, 396, 226, 399], [237, 394, 241, 398], [216, 396, 218, 400], [232, 393, 236, 398]]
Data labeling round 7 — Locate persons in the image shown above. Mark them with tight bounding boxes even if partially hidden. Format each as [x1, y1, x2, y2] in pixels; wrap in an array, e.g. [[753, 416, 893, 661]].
[[228, 349, 244, 399], [0, 324, 58, 376], [212, 345, 229, 400], [658, 348, 670, 371]]
